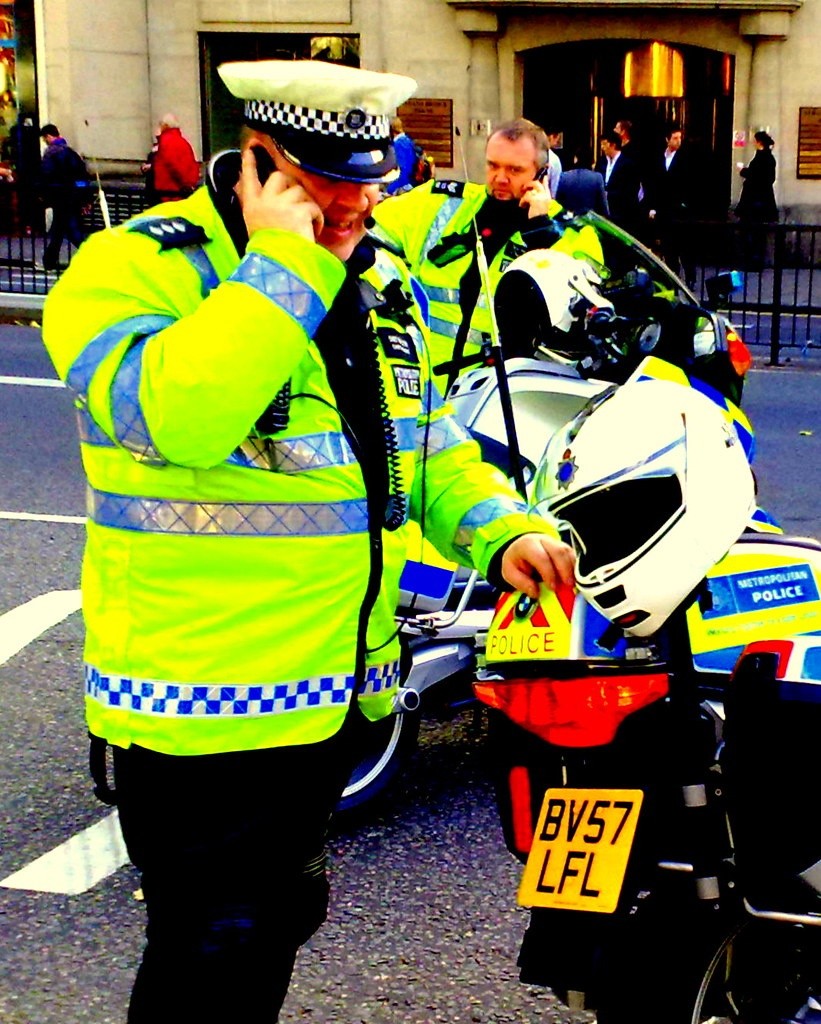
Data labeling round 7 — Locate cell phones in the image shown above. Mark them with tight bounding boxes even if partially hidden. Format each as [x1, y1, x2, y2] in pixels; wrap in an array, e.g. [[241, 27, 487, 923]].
[[533, 167, 548, 183], [249, 145, 277, 188]]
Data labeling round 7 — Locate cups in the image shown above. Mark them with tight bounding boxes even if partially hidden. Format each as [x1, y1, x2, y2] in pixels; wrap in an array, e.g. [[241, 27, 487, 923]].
[[737, 162, 743, 174]]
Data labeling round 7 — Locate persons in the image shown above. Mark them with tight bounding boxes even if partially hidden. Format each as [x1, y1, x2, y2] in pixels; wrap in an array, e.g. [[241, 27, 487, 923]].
[[43, 59, 580, 1023], [1, 116, 780, 398]]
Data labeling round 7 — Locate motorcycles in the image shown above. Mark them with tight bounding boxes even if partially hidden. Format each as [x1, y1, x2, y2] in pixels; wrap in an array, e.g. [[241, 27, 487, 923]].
[[331, 211, 761, 814], [475, 354, 820, 1024]]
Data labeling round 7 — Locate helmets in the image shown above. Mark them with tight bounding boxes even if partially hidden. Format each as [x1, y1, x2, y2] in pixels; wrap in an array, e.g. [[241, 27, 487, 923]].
[[493, 249, 599, 342], [526, 380, 762, 640]]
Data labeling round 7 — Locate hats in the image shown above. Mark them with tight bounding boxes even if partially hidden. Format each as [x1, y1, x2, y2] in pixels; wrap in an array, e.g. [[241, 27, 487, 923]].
[[217, 61, 416, 184]]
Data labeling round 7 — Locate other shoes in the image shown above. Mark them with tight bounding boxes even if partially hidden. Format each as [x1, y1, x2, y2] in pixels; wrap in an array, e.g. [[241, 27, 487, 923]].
[[34, 262, 56, 275]]
